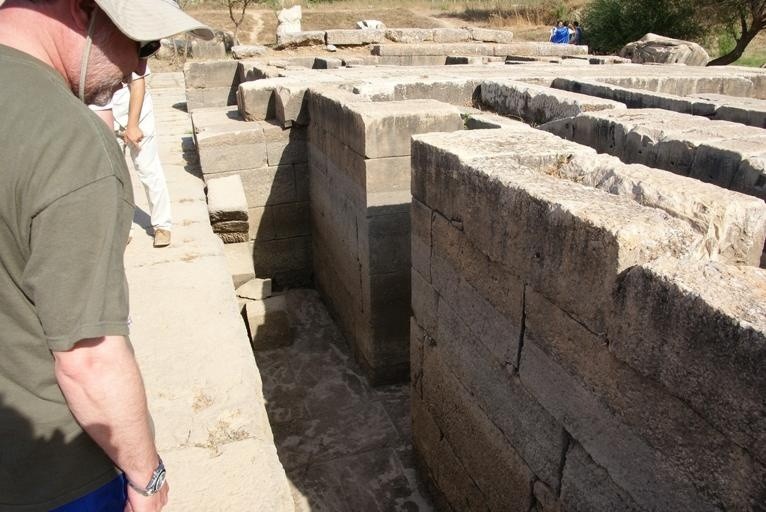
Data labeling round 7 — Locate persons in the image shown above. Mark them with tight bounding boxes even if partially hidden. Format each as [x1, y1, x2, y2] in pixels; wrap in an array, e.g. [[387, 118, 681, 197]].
[[0, 0, 215, 512], [568, 20, 581, 45], [550, 19, 575, 44], [549, 19, 564, 39], [86, 55, 171, 251], [567, 23, 575, 34]]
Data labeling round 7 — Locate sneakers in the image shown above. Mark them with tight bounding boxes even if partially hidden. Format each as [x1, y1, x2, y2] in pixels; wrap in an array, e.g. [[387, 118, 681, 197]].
[[153, 228, 172, 247]]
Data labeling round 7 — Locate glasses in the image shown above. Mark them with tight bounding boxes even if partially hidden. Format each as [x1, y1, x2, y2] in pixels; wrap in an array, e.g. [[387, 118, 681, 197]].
[[139, 39, 164, 60]]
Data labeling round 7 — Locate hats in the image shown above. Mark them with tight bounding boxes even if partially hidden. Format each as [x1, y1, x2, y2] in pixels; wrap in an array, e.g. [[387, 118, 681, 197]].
[[95, 0, 215, 43]]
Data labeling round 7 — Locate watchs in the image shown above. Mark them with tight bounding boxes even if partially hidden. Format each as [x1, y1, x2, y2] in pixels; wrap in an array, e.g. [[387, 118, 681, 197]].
[[124, 456, 168, 500]]
